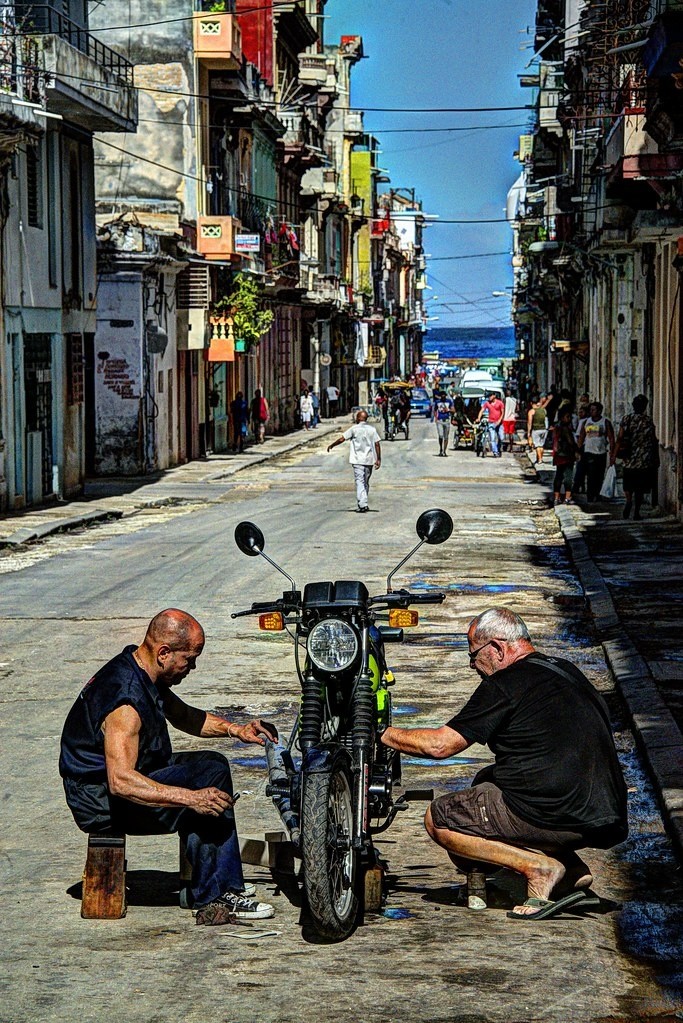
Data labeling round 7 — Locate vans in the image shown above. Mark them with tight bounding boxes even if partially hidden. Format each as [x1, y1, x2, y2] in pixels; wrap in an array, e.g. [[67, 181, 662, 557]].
[[441, 370, 506, 409]]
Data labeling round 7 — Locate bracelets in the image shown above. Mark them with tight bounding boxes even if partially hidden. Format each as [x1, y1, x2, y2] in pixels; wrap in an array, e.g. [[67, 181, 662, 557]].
[[228, 723, 238, 737]]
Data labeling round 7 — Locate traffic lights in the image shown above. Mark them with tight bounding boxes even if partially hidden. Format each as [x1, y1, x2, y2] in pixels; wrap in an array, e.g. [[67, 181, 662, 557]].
[[234, 339, 247, 353]]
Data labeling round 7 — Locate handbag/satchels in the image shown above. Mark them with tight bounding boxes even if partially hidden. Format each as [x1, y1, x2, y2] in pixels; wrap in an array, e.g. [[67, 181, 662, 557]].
[[600, 465, 617, 498]]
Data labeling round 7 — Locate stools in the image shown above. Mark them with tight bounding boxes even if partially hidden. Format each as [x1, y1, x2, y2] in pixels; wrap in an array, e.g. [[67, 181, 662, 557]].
[[81, 834, 128, 916]]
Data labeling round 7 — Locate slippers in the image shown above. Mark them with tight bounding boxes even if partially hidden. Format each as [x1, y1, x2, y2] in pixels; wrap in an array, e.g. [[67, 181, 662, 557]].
[[567, 897, 599, 909], [506, 890, 586, 920]]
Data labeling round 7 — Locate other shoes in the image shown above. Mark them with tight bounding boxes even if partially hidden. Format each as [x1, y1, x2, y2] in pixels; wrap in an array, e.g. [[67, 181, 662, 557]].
[[359, 506, 368, 512], [623, 501, 632, 519], [633, 514, 643, 520]]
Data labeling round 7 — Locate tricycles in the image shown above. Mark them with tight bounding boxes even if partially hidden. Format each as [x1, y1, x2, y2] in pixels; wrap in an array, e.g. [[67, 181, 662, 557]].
[[450, 387, 492, 458], [379, 382, 414, 442]]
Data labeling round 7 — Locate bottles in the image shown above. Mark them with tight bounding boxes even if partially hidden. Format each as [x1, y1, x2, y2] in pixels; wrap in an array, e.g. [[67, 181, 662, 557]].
[[467, 868, 487, 910]]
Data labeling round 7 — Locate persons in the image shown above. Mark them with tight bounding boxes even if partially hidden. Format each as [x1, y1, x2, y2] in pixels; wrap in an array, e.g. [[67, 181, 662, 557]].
[[552, 393, 615, 506], [394, 370, 402, 382], [229, 391, 250, 454], [502, 389, 519, 452], [327, 411, 381, 512], [610, 393, 655, 520], [59, 608, 279, 918], [374, 384, 386, 409], [446, 382, 457, 397], [452, 396, 468, 438], [250, 389, 271, 444], [475, 391, 504, 458], [408, 362, 442, 390], [433, 391, 455, 457], [300, 381, 340, 430], [507, 369, 539, 412], [524, 382, 562, 464], [381, 388, 413, 428], [377, 605, 630, 920]]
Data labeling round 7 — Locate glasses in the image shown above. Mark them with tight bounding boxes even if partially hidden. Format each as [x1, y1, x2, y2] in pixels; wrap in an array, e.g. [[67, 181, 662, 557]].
[[467, 639, 507, 662]]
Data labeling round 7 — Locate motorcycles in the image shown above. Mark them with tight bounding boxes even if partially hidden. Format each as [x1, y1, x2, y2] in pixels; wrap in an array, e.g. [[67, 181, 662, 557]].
[[231, 507, 454, 943]]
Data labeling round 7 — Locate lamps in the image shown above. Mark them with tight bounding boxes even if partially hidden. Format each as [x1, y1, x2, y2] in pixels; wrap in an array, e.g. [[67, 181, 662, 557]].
[[207, 165, 222, 193]]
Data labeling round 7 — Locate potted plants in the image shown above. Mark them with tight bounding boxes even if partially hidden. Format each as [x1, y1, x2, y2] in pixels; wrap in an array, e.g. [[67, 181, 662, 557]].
[[213, 272, 276, 351]]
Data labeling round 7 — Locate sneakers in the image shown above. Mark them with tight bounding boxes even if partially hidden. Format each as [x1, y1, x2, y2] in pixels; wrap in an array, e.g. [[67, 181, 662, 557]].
[[213, 891, 275, 919], [238, 883, 256, 897]]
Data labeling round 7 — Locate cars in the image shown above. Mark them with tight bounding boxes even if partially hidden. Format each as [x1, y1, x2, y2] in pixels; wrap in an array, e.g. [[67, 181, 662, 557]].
[[409, 388, 433, 419]]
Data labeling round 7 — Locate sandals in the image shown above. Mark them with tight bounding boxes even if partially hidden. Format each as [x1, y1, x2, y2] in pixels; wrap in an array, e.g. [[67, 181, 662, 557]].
[[553, 500, 562, 504], [564, 497, 580, 504]]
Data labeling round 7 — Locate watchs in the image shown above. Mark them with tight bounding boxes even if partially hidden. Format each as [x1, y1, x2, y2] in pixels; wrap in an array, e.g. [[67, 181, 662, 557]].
[[377, 724, 388, 739]]
